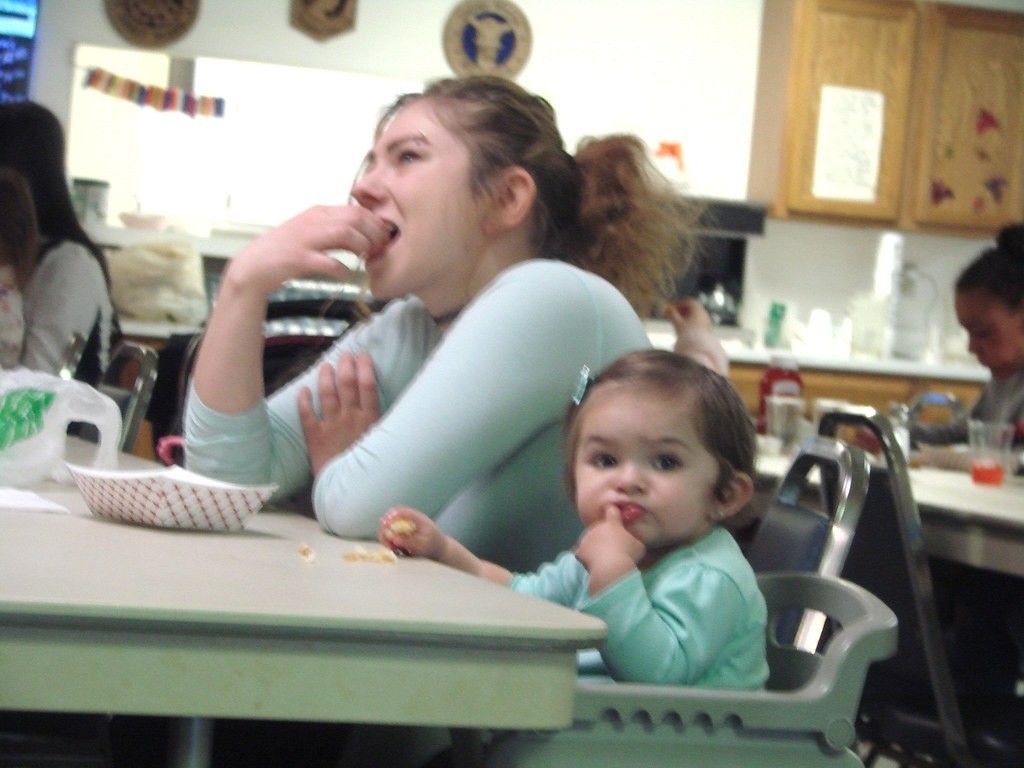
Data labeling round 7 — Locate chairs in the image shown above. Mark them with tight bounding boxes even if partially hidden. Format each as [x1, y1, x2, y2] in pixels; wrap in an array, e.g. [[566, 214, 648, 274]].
[[815, 399, 1023, 767], [740, 433, 872, 655]]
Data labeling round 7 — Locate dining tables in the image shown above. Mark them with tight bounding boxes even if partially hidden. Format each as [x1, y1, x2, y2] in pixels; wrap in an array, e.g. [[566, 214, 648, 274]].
[[1, 433, 611, 768], [745, 430, 1024, 575]]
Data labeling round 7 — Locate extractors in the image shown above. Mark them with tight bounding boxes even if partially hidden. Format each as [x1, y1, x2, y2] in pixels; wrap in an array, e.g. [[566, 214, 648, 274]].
[[671, 199, 768, 241]]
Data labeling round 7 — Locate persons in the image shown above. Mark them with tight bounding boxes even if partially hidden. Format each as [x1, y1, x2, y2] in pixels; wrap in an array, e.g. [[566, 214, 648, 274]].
[[375, 349, 771, 681], [904, 223, 1024, 482], [0, 165, 38, 368], [1, 97, 123, 443], [184, 75, 706, 570]]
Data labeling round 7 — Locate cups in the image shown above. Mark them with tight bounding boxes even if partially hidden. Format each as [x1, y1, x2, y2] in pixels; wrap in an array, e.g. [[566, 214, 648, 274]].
[[767, 393, 909, 470], [965, 418, 1014, 488]]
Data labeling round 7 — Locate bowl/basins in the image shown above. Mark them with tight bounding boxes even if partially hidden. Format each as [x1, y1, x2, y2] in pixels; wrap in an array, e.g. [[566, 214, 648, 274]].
[[119, 212, 169, 232]]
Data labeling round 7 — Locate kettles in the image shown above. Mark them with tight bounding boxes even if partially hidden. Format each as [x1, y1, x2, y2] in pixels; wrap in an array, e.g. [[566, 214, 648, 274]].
[[697, 282, 737, 322]]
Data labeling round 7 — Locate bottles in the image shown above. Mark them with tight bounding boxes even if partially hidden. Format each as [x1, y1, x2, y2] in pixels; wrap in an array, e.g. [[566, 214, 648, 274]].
[[756, 354, 803, 436]]
